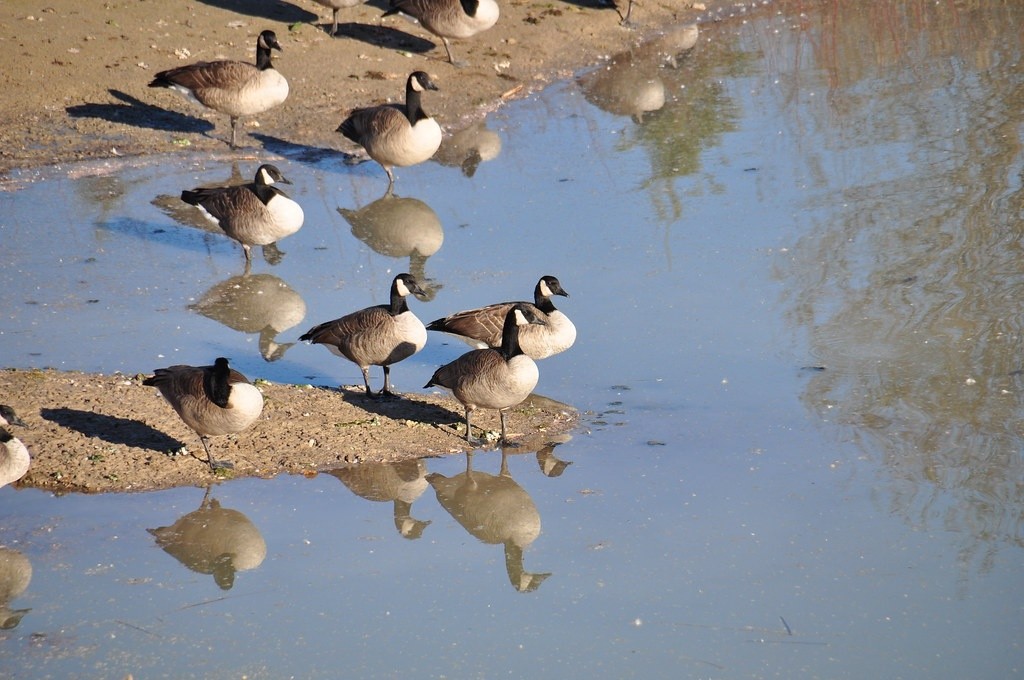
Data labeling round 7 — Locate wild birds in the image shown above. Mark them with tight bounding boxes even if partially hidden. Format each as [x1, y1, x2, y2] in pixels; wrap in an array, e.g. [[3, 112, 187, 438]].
[[423, 441, 577, 594], [148, 28, 290, 149], [326, 457, 434, 541], [427, 117, 502, 178], [335, 183, 446, 303], [145, 488, 268, 591], [142, 358, 264, 468], [0, 404, 30, 492], [0, 544, 34, 631], [419, 276, 578, 443], [179, 162, 304, 267], [332, 69, 443, 184], [181, 267, 307, 364], [582, 23, 701, 129], [298, 271, 428, 404], [318, 0, 501, 67]]
